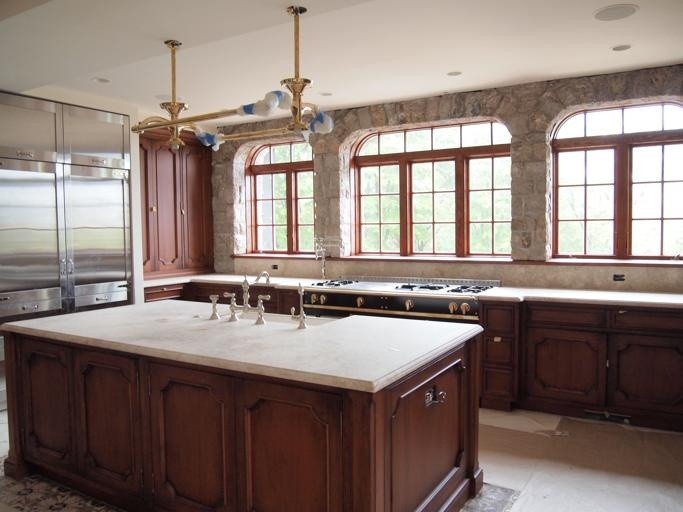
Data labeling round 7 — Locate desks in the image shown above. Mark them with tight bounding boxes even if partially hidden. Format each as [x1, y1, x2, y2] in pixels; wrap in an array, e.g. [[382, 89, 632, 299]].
[[0, 298, 485, 512]]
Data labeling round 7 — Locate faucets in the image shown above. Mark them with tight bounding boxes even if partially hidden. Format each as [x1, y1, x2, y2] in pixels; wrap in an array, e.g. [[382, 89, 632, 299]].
[[254, 271, 269, 284]]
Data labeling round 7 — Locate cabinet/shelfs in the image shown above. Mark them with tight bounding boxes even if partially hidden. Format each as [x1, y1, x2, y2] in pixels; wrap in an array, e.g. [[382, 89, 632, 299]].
[[144, 282, 188, 303], [521, 299, 683, 430], [63, 163, 135, 308], [140, 132, 212, 274], [61, 100, 132, 169], [1, 91, 61, 163], [1, 161, 69, 313], [195, 283, 298, 315], [476, 300, 520, 411]]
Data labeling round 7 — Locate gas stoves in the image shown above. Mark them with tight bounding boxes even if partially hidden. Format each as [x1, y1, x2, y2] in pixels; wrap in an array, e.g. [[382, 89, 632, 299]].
[[302, 278, 494, 320]]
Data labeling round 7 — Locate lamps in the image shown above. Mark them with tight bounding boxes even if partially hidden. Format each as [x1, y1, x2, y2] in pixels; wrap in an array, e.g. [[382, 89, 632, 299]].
[[133, 5, 336, 151]]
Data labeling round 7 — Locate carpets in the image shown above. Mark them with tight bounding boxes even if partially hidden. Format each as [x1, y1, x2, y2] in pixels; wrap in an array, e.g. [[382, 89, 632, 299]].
[[0, 454, 523, 512]]
